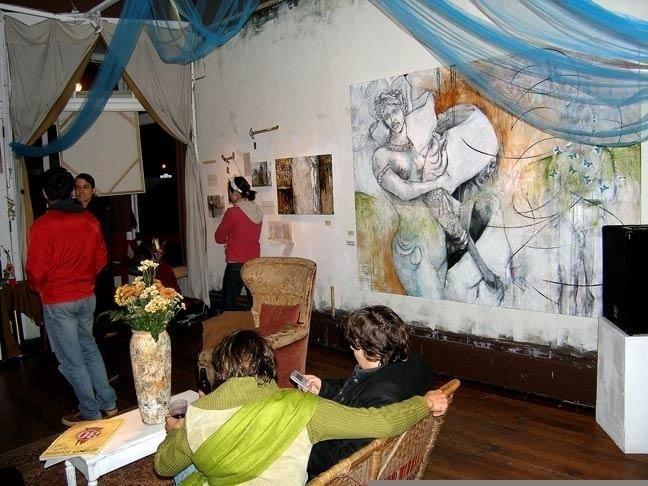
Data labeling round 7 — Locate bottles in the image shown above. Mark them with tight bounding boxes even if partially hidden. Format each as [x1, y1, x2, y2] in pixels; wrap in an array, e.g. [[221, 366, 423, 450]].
[[197, 368, 211, 397]]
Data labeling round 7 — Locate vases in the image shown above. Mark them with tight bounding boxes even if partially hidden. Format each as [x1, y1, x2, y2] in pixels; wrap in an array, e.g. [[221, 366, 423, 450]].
[[128, 328, 173, 426]]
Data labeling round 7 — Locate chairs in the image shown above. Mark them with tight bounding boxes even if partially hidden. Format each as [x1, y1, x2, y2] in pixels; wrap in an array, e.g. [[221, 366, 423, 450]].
[[197, 253, 319, 393], [373, 376, 459, 480], [308, 442, 381, 486]]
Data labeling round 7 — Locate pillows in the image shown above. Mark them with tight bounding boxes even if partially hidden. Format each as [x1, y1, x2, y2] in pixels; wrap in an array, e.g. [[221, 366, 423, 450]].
[[257, 302, 301, 328]]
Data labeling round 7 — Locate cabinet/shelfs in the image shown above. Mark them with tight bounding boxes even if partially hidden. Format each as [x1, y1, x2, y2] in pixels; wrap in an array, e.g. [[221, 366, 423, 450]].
[[589, 315, 647, 460]]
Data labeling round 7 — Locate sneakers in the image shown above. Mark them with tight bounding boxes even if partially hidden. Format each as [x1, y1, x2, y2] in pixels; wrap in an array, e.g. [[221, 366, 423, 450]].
[[62, 410, 103, 426]]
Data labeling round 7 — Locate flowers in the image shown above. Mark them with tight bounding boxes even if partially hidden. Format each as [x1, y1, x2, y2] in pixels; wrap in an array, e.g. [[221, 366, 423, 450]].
[[99, 257, 186, 340]]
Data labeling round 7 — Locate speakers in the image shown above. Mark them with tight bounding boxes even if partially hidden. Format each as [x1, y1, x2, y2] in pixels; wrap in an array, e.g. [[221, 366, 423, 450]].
[[601, 224, 648, 336]]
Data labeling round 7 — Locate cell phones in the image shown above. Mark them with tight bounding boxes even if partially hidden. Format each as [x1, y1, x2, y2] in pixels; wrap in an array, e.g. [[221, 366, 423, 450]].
[[290, 370, 312, 390]]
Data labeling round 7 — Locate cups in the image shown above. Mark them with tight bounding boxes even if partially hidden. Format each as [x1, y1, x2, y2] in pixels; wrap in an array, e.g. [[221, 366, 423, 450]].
[[168, 399, 187, 419], [7, 277, 17, 288]]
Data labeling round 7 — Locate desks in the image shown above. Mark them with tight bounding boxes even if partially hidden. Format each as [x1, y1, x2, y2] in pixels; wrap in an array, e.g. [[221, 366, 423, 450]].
[[0, 278, 49, 369], [61, 389, 205, 486]]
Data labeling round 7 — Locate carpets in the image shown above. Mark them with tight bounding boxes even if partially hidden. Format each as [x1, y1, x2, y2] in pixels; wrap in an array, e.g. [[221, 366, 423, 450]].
[[0, 423, 181, 486]]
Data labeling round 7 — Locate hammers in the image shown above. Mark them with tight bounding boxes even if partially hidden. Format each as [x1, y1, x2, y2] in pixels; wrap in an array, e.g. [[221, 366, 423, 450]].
[[249, 125, 279, 140]]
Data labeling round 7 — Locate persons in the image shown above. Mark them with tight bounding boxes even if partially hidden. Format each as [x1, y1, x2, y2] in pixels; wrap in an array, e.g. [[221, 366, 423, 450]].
[[154, 329, 449, 486], [138, 241, 181, 296], [26, 167, 119, 427], [460, 157, 498, 234], [297, 305, 434, 483], [372, 90, 451, 301], [74, 173, 117, 337], [215, 177, 258, 311]]
